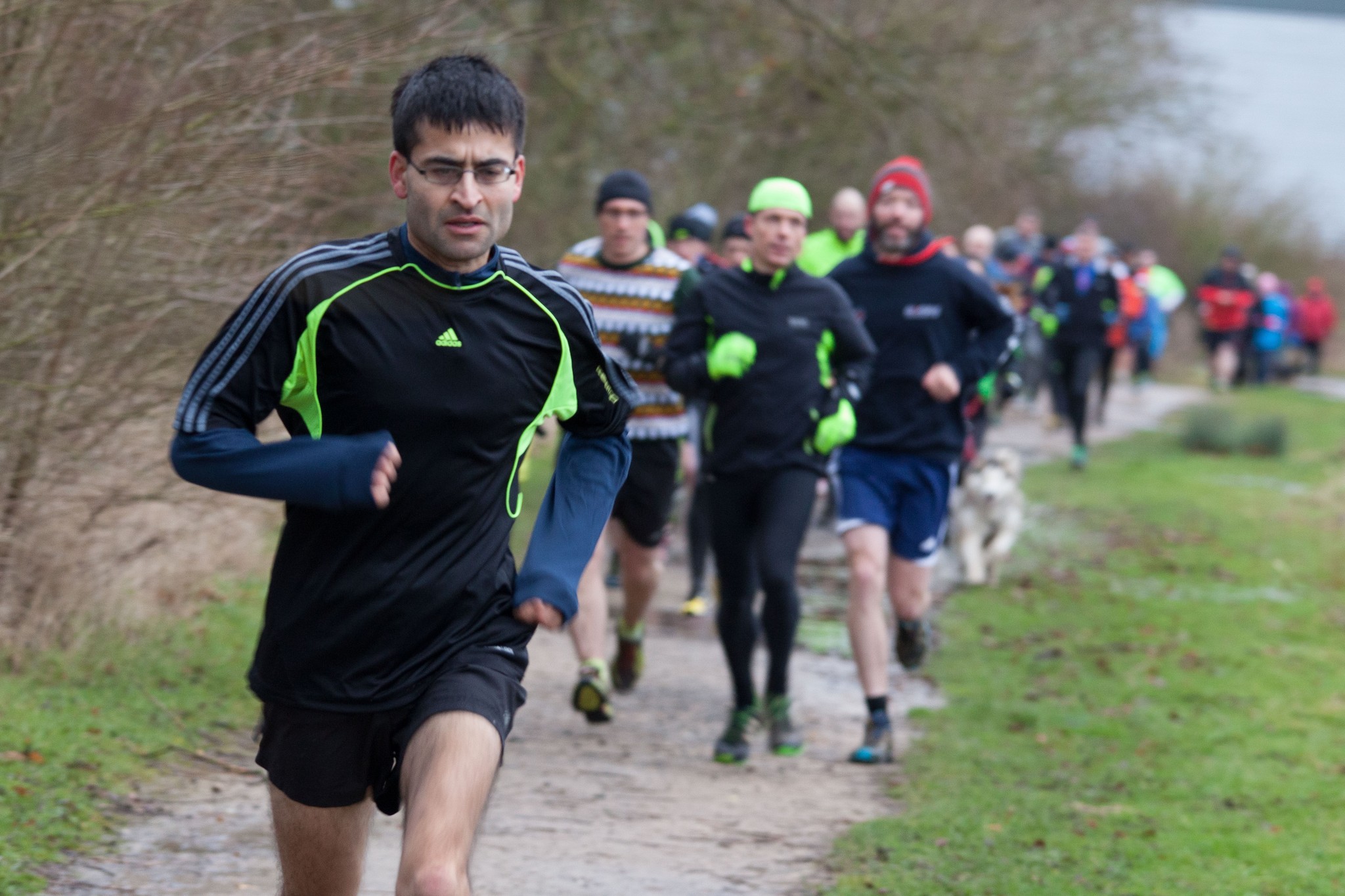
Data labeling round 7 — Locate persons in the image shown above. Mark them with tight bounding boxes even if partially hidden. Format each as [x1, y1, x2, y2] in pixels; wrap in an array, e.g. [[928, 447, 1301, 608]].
[[816, 152, 1028, 764], [556, 170, 706, 726], [604, 187, 1339, 618], [167, 52, 656, 896], [659, 176, 877, 764]]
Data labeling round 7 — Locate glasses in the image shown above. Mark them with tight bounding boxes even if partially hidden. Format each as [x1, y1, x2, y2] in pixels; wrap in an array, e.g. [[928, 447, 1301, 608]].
[[404, 153, 516, 184], [597, 207, 652, 219]]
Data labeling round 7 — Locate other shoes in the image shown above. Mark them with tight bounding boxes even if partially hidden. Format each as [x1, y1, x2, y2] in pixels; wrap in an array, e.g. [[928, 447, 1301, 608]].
[[618, 393, 1111, 616]]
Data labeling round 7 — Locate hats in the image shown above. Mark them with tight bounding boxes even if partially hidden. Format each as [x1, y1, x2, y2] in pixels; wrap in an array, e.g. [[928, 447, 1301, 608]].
[[594, 169, 654, 216], [748, 176, 813, 218], [723, 208, 757, 242], [863, 152, 957, 265], [668, 201, 721, 241]]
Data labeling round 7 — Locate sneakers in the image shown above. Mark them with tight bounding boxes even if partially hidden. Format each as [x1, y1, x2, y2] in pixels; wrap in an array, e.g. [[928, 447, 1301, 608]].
[[711, 694, 766, 764], [892, 617, 926, 666], [849, 721, 897, 763], [762, 690, 802, 757], [611, 617, 647, 696], [569, 657, 615, 723]]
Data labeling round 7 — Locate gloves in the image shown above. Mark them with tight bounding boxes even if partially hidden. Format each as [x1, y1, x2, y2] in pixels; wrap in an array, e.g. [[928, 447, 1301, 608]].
[[706, 330, 756, 381]]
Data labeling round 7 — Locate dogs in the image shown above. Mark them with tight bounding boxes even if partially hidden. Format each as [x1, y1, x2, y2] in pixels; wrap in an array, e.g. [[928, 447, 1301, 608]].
[[947, 448, 1022, 587]]
